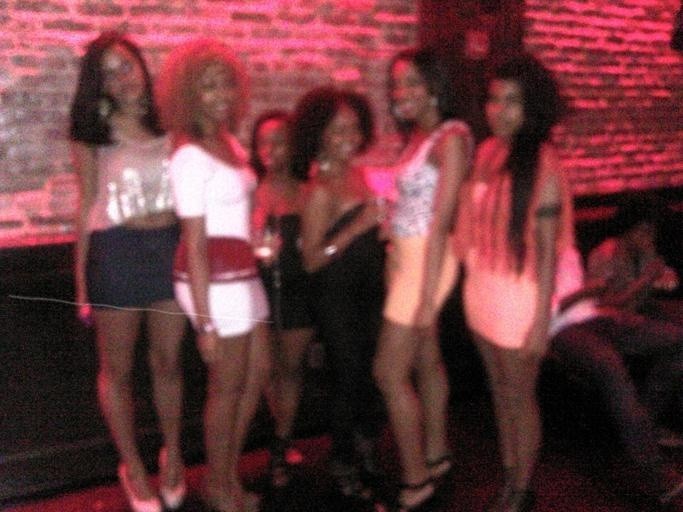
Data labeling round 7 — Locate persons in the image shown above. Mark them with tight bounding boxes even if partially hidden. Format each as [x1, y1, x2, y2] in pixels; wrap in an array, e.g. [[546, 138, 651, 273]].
[[61, 28, 682, 512]]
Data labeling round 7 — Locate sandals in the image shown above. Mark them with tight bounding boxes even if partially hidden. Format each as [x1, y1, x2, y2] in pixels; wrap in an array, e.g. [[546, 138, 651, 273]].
[[397, 480, 440, 511], [425, 453, 459, 489]]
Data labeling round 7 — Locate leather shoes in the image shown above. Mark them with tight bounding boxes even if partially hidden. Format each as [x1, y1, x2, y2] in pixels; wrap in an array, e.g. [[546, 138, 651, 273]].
[[119, 464, 163, 511], [158, 448, 189, 508]]
